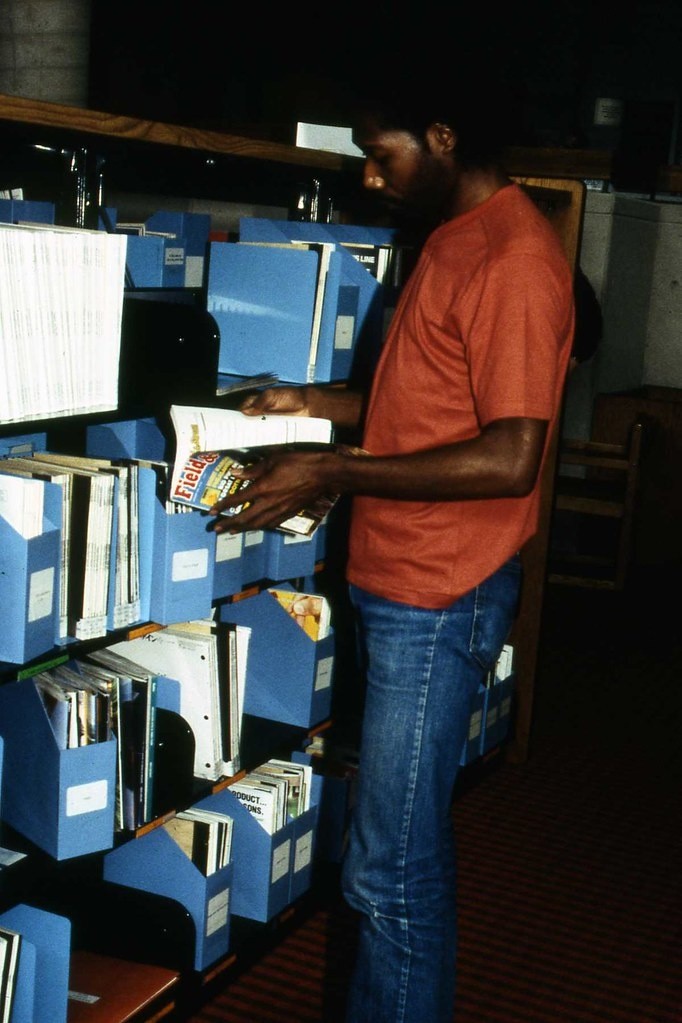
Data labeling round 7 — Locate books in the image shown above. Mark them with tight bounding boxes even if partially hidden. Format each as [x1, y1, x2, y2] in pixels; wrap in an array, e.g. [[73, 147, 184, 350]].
[[104, 619, 252, 780], [237, 238, 393, 384], [0, 452, 193, 640], [0, 187, 24, 201], [34, 650, 157, 834], [168, 404, 343, 537], [228, 758, 312, 835], [115, 221, 177, 239], [266, 586, 331, 642], [0, 927, 23, 1023], [162, 808, 234, 879], [0, 219, 126, 422], [481, 636, 523, 690]]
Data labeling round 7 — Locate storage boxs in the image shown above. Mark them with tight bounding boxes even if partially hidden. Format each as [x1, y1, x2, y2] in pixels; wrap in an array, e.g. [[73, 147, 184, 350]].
[[1, 188, 399, 1023]]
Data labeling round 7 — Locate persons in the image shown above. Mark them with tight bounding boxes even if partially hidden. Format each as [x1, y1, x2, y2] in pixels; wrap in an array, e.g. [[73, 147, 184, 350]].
[[213, 62, 577, 1023]]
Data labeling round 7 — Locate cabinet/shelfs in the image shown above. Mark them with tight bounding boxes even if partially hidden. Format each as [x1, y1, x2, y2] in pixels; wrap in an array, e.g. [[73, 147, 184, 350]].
[[0, 90, 585, 1023], [549, 411, 642, 592]]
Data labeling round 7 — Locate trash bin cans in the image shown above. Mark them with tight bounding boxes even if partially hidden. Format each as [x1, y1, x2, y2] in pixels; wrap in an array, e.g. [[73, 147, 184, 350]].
[[592, 383, 681, 567]]
